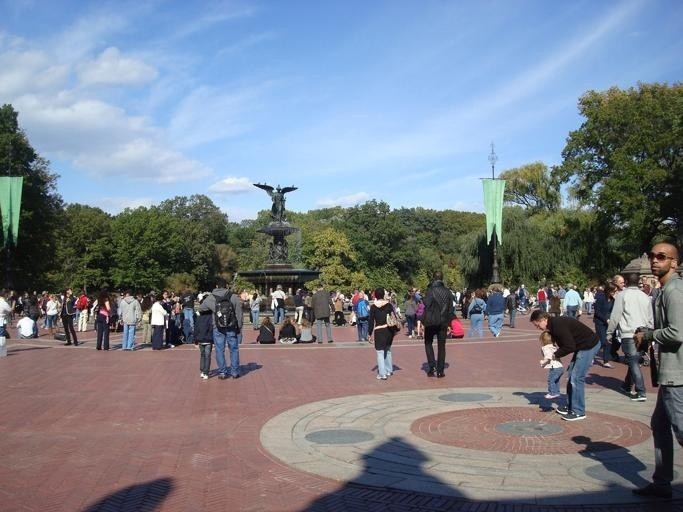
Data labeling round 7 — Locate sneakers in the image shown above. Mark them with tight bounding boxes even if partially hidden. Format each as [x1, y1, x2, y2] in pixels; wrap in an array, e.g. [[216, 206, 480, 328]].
[[427, 365, 446, 377], [620, 385, 632, 393], [603, 363, 615, 369], [318, 341, 333, 343], [376, 372, 391, 380], [199, 372, 239, 380], [556, 406, 585, 421], [632, 482, 672, 498], [544, 393, 559, 400], [630, 394, 647, 401]]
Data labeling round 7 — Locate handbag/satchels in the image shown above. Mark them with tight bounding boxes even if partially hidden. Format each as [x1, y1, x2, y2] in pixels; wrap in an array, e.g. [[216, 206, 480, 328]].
[[386, 311, 401, 332], [470, 304, 482, 314], [54, 331, 66, 341], [93, 304, 100, 313]]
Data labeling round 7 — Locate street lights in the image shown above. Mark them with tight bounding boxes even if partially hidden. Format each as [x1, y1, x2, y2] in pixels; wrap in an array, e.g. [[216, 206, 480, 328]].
[[488, 140, 502, 284]]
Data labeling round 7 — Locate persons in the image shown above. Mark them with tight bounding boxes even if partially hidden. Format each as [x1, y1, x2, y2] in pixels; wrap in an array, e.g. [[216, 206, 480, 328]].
[[583, 275, 654, 400], [633, 242, 683, 498], [367, 288, 397, 379], [1, 288, 92, 347], [530, 310, 602, 421], [421, 271, 453, 377], [194, 278, 243, 380], [95, 291, 142, 351], [528, 294, 536, 310], [141, 287, 198, 350], [312, 284, 528, 346], [249, 284, 317, 344], [537, 281, 583, 321]]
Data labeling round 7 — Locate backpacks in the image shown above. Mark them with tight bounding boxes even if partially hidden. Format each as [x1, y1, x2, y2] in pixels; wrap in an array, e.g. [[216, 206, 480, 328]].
[[357, 302, 369, 318], [216, 292, 237, 333], [422, 296, 441, 326]]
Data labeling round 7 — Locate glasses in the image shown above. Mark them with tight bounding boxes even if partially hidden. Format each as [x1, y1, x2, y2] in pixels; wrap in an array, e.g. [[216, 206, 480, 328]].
[[648, 252, 673, 263]]
